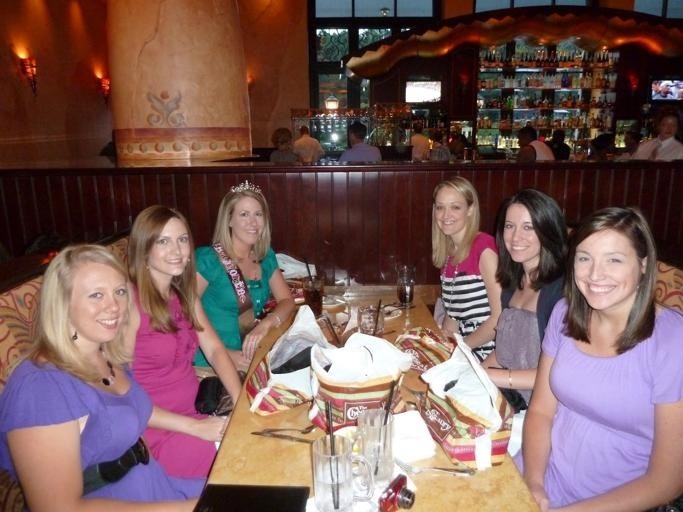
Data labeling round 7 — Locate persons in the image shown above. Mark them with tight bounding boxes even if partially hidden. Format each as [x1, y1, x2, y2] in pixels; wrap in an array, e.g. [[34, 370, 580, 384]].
[[632, 114, 683, 162], [590, 134, 616, 159], [652, 80, 683, 100], [430, 175, 503, 364], [506, 207, 683, 512], [270, 128, 303, 164], [411, 122, 429, 161], [427, 131, 451, 162], [623, 127, 645, 155], [338, 122, 382, 162], [515, 127, 555, 161], [125, 206, 244, 480], [479, 188, 568, 413], [189, 188, 297, 381], [0, 243, 198, 512], [292, 125, 324, 164], [545, 128, 571, 160]]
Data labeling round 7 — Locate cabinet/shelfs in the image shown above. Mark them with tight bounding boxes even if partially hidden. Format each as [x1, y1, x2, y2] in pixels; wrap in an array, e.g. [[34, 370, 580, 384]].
[[475, 41, 620, 153]]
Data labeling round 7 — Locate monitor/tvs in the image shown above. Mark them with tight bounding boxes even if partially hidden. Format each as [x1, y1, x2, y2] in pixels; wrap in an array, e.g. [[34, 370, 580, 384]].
[[403, 77, 443, 104], [648, 79, 683, 101]]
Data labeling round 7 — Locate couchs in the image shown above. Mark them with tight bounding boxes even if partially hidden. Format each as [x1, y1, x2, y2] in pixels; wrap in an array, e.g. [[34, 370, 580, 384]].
[[566, 228, 683, 314], [0, 228, 130, 512]]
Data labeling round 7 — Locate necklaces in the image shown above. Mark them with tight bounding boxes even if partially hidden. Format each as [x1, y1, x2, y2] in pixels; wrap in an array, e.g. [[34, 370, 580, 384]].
[[440, 249, 459, 314], [246, 260, 258, 296], [86, 347, 116, 386]]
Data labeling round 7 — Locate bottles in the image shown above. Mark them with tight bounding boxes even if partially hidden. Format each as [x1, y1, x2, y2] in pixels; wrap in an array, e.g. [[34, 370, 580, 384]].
[[475, 42, 621, 149]]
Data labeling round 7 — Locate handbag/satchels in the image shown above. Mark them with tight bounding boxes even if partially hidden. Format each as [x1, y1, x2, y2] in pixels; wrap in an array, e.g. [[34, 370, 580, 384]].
[[195, 370, 246, 416]]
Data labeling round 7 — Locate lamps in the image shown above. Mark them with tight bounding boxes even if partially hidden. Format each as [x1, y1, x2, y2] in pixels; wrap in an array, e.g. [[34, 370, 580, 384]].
[[100, 78, 110, 107], [323, 95, 339, 113], [20, 58, 37, 95]]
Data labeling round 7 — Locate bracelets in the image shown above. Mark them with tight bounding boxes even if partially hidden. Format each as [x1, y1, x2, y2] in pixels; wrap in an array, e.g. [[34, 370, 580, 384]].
[[266, 312, 281, 327], [508, 368, 512, 390]]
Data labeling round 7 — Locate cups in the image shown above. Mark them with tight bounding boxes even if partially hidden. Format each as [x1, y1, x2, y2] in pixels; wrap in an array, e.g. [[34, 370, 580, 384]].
[[396, 270, 416, 306], [302, 279, 324, 317], [356, 306, 383, 334], [312, 435, 375, 512], [351, 409, 392, 480], [464, 148, 475, 163], [448, 152, 455, 164]]
